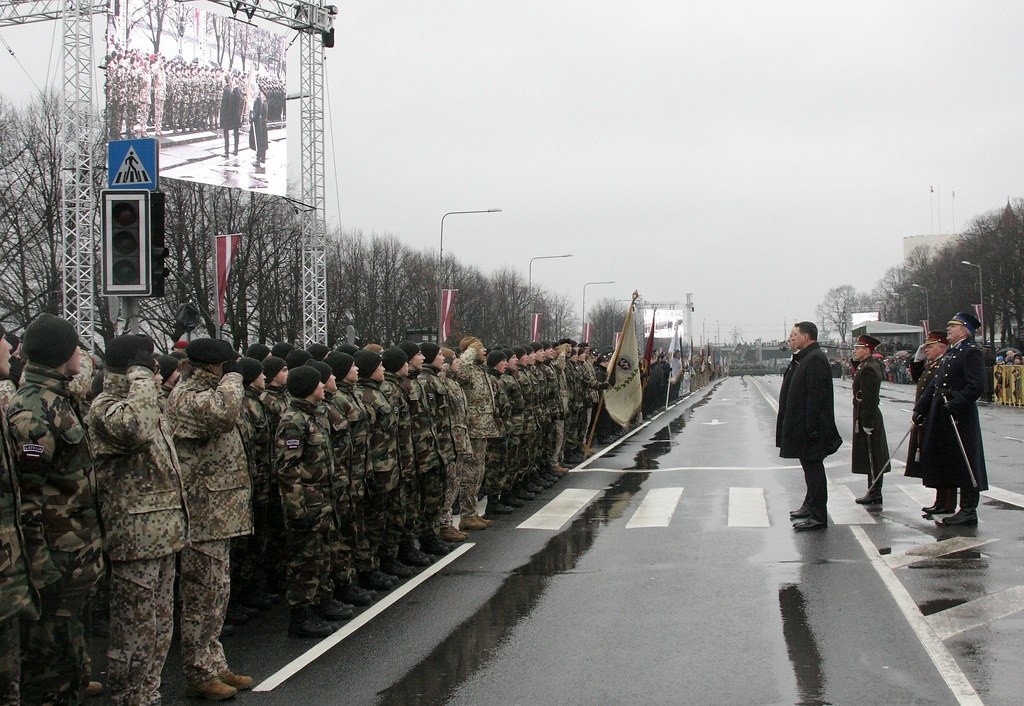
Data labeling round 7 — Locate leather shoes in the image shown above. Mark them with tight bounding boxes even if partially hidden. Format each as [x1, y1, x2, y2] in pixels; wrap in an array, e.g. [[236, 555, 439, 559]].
[[790, 504, 810, 517], [922, 504, 954, 515], [792, 509, 827, 530]]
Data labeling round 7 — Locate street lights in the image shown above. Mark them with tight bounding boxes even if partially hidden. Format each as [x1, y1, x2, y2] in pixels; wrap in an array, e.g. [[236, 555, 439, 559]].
[[438, 209, 505, 345], [960, 261, 986, 346], [527, 254, 575, 313], [876, 300, 888, 323], [612, 298, 643, 351], [716, 320, 721, 349], [892, 291, 910, 325], [911, 283, 930, 335], [580, 280, 619, 346], [702, 318, 706, 348]]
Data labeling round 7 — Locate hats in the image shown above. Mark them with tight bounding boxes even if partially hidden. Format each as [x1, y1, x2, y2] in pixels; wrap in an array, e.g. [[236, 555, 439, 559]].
[[853, 332, 881, 352], [924, 327, 950, 345], [22, 311, 78, 369], [107, 338, 623, 395], [946, 311, 981, 336]]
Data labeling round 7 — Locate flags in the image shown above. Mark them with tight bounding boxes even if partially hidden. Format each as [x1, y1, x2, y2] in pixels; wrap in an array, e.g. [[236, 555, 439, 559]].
[[668, 327, 682, 381], [688, 342, 693, 362], [601, 306, 642, 428], [700, 348, 704, 362], [644, 319, 654, 376], [707, 344, 711, 367]]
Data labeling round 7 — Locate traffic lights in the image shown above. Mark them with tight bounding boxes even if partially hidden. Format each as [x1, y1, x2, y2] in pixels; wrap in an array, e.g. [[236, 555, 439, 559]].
[[148, 190, 172, 301], [102, 188, 154, 297]]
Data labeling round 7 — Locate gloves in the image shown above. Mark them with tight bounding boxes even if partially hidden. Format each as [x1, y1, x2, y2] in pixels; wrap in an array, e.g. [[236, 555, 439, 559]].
[[127, 351, 156, 372], [220, 359, 244, 376], [944, 400, 957, 418], [912, 409, 924, 425], [468, 340, 481, 352], [41, 582, 58, 620], [862, 426, 874, 435], [292, 516, 308, 532], [914, 344, 927, 363]]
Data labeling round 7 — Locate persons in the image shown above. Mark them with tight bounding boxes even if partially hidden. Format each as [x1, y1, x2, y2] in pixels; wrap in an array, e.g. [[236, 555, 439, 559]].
[[774, 321, 843, 529], [107, 34, 286, 167], [851, 335, 892, 504], [0, 312, 722, 706], [903, 311, 989, 526], [779, 342, 1024, 406]]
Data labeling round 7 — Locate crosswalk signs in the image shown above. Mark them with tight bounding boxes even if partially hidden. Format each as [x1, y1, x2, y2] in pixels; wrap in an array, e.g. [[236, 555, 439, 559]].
[[106, 138, 159, 190]]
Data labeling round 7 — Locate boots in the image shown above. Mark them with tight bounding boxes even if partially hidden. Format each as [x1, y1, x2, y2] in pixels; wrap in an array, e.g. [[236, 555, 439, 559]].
[[942, 486, 977, 526], [87, 420, 639, 706], [855, 471, 882, 504]]
